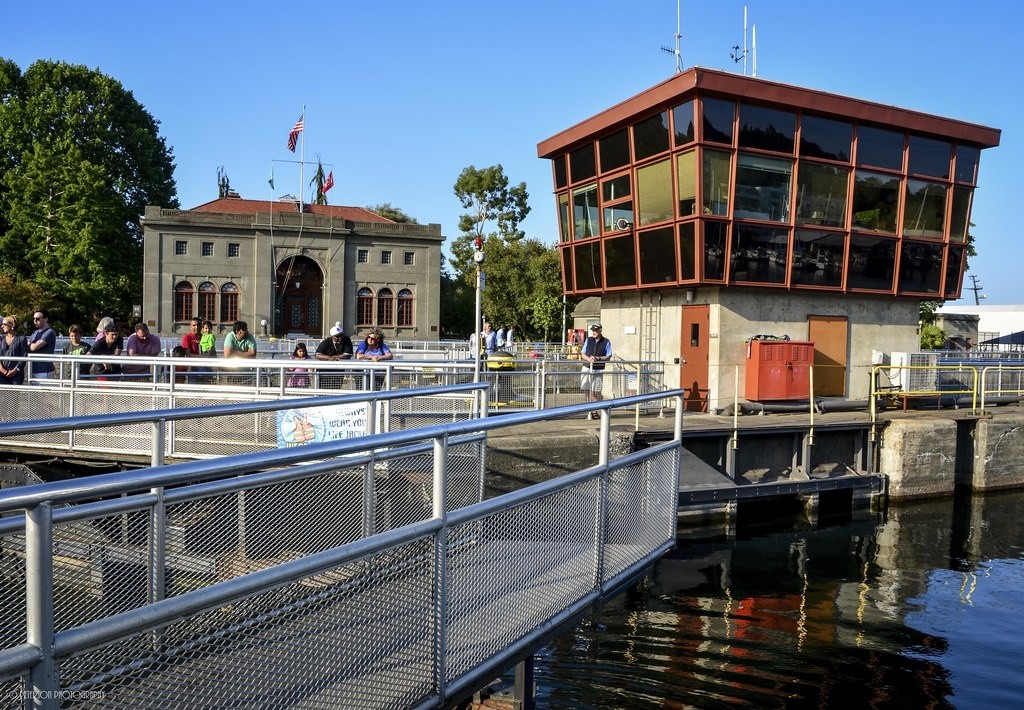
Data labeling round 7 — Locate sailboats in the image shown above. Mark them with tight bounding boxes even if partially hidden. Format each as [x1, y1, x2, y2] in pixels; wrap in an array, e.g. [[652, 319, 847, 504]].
[[705, 183, 942, 272]]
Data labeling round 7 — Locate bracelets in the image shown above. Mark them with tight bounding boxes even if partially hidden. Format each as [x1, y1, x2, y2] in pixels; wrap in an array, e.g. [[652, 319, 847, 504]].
[[598, 355, 600, 360]]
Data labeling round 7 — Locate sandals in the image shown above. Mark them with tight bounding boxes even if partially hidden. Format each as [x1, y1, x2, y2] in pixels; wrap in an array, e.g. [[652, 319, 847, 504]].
[[591, 412, 600, 419]]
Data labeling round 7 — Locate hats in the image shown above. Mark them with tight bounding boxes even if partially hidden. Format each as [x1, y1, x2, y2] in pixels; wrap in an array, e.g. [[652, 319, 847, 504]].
[[589, 323, 602, 331], [104, 325, 119, 332], [329, 321, 343, 336]]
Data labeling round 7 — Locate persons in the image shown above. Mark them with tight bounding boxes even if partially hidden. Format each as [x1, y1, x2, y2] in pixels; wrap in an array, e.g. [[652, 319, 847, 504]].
[[570, 330, 580, 345], [0, 314, 27, 421], [356, 327, 393, 392], [121, 323, 161, 382], [580, 324, 612, 420], [63, 324, 91, 387], [224, 320, 257, 384], [182, 317, 217, 385], [496, 324, 506, 351], [315, 321, 353, 389], [507, 325, 516, 348], [85, 317, 123, 388], [469, 322, 496, 372], [27, 309, 56, 385], [161, 346, 188, 384], [59, 332, 63, 337], [286, 342, 314, 388]]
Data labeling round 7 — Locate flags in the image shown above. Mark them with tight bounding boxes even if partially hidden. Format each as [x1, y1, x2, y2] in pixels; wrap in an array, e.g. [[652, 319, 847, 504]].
[[288, 113, 304, 153], [322, 171, 334, 192]]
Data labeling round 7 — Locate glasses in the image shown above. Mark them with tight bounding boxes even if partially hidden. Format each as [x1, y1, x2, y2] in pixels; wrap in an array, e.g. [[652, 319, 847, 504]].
[[335, 334, 341, 338], [1, 323, 6, 326], [33, 317, 41, 321], [368, 335, 375, 340]]
